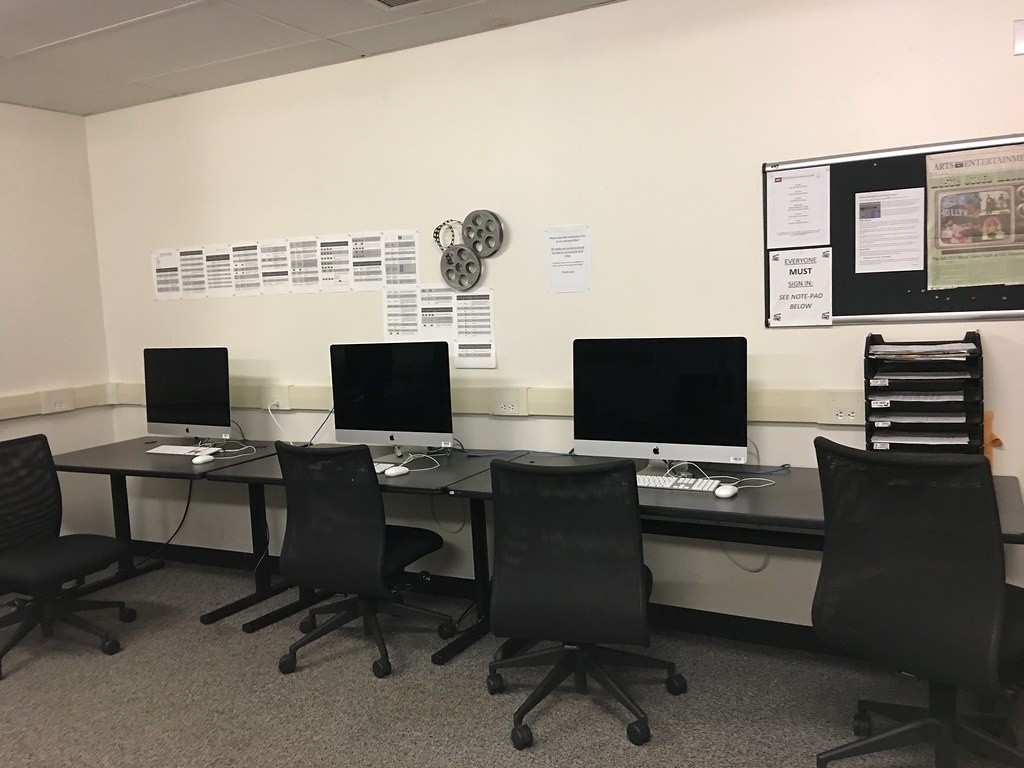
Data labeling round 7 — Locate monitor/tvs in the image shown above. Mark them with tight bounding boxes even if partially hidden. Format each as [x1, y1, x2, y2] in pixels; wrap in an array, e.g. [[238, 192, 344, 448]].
[[573, 337, 748, 478], [331, 341, 454, 464], [144, 347, 231, 446]]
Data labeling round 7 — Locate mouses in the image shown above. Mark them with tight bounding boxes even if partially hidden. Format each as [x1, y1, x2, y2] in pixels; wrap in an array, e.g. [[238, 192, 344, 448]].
[[715, 485, 738, 498], [192, 454, 214, 465], [385, 466, 410, 476]]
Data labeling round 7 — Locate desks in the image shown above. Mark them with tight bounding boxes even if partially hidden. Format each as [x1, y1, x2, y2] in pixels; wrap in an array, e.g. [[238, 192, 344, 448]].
[[447, 452, 1023, 663], [208, 440, 528, 666], [55, 434, 312, 626]]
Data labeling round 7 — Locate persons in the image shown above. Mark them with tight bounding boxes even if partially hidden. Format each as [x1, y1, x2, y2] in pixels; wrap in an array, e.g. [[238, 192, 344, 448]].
[[982, 217, 1007, 240], [986, 193, 1005, 211]]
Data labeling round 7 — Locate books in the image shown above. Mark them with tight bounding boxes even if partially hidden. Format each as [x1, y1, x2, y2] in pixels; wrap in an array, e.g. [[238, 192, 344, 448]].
[[146, 444, 222, 456]]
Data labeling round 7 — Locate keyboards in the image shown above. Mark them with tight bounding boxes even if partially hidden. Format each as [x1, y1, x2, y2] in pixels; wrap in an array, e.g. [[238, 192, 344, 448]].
[[637, 475, 721, 492], [308, 460, 395, 473], [146, 445, 222, 456]]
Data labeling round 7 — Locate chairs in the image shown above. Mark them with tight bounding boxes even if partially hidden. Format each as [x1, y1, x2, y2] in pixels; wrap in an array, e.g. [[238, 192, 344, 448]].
[[1, 433, 137, 662], [485, 460, 687, 749], [271, 440, 454, 675], [812, 437, 1024, 768]]
